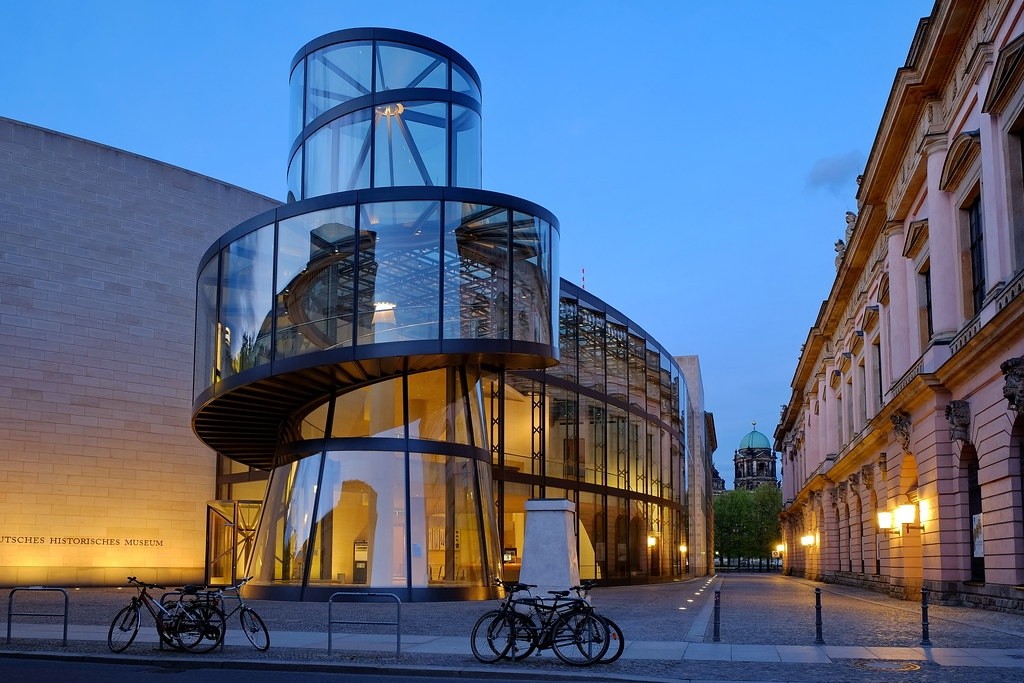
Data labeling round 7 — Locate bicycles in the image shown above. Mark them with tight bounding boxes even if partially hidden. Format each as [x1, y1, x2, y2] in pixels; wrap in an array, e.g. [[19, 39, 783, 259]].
[[158, 577, 271, 652], [107, 576, 227, 653], [488, 579, 626, 663], [471, 576, 611, 664]]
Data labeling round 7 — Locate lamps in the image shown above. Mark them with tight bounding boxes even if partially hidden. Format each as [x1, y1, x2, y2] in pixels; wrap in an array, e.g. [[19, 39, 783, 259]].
[[896, 504, 925, 533], [878, 511, 900, 538]]
